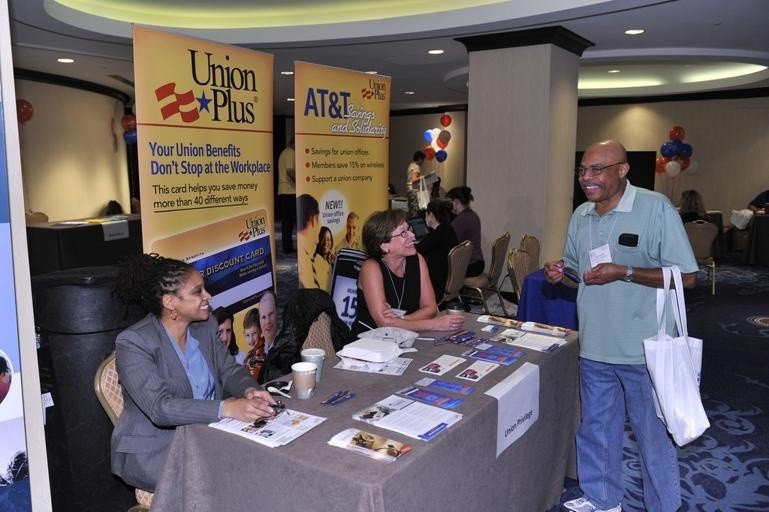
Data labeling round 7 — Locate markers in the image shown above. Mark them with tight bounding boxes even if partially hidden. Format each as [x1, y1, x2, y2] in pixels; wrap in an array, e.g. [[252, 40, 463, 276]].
[[553, 264, 582, 283], [320, 389, 356, 405], [443, 329, 488, 347]]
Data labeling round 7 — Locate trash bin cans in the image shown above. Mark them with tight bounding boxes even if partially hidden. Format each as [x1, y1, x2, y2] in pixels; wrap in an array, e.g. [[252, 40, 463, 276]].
[[31, 266, 149, 469]]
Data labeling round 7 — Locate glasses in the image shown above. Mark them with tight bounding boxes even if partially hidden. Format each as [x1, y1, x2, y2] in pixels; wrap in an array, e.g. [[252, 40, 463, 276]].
[[392, 225, 414, 238], [575, 162, 625, 176]]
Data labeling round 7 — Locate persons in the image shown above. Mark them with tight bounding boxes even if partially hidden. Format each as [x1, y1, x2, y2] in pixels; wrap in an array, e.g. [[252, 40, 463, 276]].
[[277, 131, 296, 256], [349, 209, 465, 342], [296, 194, 358, 293], [678, 186, 710, 226], [109, 262, 279, 492], [542, 135, 699, 512], [747, 189, 769, 215], [211, 290, 278, 383], [406, 149, 486, 312]]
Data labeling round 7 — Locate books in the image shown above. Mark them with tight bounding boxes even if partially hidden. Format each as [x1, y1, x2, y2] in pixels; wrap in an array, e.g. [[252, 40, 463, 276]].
[[207, 406, 327, 450], [53, 213, 128, 227], [327, 307, 571, 468]]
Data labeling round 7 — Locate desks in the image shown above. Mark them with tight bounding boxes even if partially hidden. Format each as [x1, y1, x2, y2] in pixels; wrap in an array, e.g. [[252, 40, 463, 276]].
[[739, 214, 769, 267], [515, 268, 579, 332], [148, 307, 585, 512], [25, 212, 142, 278]]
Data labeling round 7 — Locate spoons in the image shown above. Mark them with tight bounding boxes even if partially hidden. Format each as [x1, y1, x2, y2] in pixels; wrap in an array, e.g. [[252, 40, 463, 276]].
[[267, 386, 291, 400]]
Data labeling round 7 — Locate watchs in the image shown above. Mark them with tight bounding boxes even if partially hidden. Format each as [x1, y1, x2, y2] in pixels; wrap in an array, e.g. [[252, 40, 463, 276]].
[[624, 265, 635, 283]]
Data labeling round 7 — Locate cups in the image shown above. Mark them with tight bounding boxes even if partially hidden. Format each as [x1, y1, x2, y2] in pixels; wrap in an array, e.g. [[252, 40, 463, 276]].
[[290, 362, 319, 401], [446, 301, 464, 317], [301, 348, 327, 382]]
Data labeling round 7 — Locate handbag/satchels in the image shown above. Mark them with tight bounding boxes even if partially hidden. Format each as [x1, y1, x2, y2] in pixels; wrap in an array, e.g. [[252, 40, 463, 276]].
[[643, 333, 711, 447], [417, 191, 430, 210]]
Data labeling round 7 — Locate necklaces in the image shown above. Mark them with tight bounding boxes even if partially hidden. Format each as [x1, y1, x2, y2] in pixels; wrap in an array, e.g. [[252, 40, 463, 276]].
[[382, 259, 406, 310]]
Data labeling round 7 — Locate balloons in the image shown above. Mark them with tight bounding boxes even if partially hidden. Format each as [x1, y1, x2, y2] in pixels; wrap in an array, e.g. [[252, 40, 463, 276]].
[[423, 113, 453, 163], [655, 123, 692, 182]]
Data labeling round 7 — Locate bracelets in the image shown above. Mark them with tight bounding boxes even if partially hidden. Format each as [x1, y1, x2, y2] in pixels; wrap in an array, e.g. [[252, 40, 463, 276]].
[[400, 313, 409, 320]]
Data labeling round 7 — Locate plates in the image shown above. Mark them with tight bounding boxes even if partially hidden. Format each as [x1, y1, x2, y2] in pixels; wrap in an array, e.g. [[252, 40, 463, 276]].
[[266, 382, 290, 395]]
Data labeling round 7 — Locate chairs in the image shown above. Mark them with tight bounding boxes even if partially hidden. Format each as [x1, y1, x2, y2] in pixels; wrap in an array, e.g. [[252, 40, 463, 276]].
[[683, 219, 720, 296], [90, 347, 154, 512], [282, 287, 357, 359], [436, 231, 541, 319]]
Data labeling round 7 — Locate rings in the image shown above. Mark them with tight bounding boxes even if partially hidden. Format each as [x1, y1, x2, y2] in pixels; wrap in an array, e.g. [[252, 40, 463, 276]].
[[452, 323, 456, 327]]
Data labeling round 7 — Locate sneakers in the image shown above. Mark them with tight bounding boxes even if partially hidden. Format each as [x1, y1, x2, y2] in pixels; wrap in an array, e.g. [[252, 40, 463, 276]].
[[562, 496, 621, 512]]
